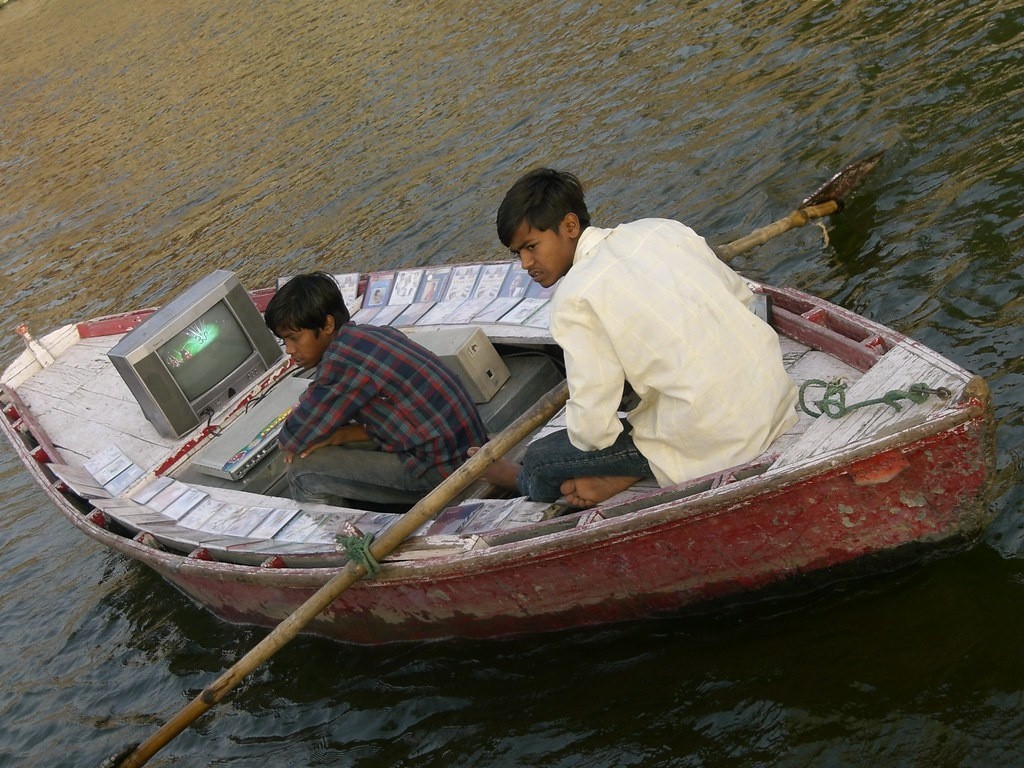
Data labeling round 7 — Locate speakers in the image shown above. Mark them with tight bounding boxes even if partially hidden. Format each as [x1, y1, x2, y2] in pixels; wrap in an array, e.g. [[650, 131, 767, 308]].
[[408, 326, 512, 404]]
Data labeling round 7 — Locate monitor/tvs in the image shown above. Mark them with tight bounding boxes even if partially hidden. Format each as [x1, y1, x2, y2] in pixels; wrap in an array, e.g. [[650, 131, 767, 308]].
[[106, 269, 284, 441]]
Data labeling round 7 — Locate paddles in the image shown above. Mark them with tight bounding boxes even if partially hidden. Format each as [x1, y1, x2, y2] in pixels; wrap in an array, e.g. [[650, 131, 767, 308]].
[[93, 150, 888, 768]]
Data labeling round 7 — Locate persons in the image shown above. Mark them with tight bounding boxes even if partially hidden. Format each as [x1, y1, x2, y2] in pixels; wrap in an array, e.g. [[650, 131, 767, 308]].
[[465, 168, 799, 508], [264, 270, 489, 507]]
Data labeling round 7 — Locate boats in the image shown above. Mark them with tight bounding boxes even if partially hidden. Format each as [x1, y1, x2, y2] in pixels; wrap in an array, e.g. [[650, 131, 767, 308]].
[[0, 259, 1003, 649]]
